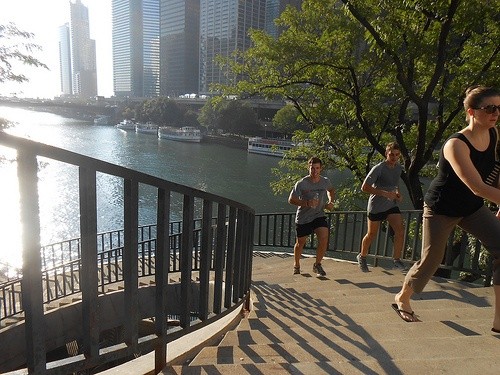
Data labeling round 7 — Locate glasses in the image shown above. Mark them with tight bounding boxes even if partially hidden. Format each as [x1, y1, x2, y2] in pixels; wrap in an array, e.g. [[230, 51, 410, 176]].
[[472, 104, 500, 113]]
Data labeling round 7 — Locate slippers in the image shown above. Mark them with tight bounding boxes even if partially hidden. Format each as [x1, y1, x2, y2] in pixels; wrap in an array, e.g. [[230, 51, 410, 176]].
[[491, 328, 500, 332], [391, 303, 417, 321]]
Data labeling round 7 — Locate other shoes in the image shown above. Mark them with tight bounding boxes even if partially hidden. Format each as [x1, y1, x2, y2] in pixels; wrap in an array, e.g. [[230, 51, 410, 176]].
[[392, 258, 404, 266], [313, 262, 326, 277], [293, 265, 300, 273], [357, 253, 368, 271]]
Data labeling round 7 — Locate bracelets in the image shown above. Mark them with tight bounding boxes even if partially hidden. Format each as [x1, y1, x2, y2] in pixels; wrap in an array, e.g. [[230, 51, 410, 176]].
[[330, 202, 334, 205], [307, 200, 309, 206]]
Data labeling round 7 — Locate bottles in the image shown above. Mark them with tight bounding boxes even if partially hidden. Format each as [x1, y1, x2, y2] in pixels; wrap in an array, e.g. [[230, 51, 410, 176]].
[[386, 186, 399, 200], [311, 193, 319, 209]]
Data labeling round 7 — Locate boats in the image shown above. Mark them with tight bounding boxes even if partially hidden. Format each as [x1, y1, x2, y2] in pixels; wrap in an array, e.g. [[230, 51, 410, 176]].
[[94, 117, 108, 125], [116, 119, 136, 130], [246, 137, 313, 160], [135, 121, 160, 134], [157, 126, 200, 143]]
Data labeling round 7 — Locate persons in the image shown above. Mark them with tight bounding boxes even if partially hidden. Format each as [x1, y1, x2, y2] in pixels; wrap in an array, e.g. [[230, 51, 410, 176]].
[[356, 142, 409, 271], [395, 86, 500, 333], [288, 157, 334, 278]]
